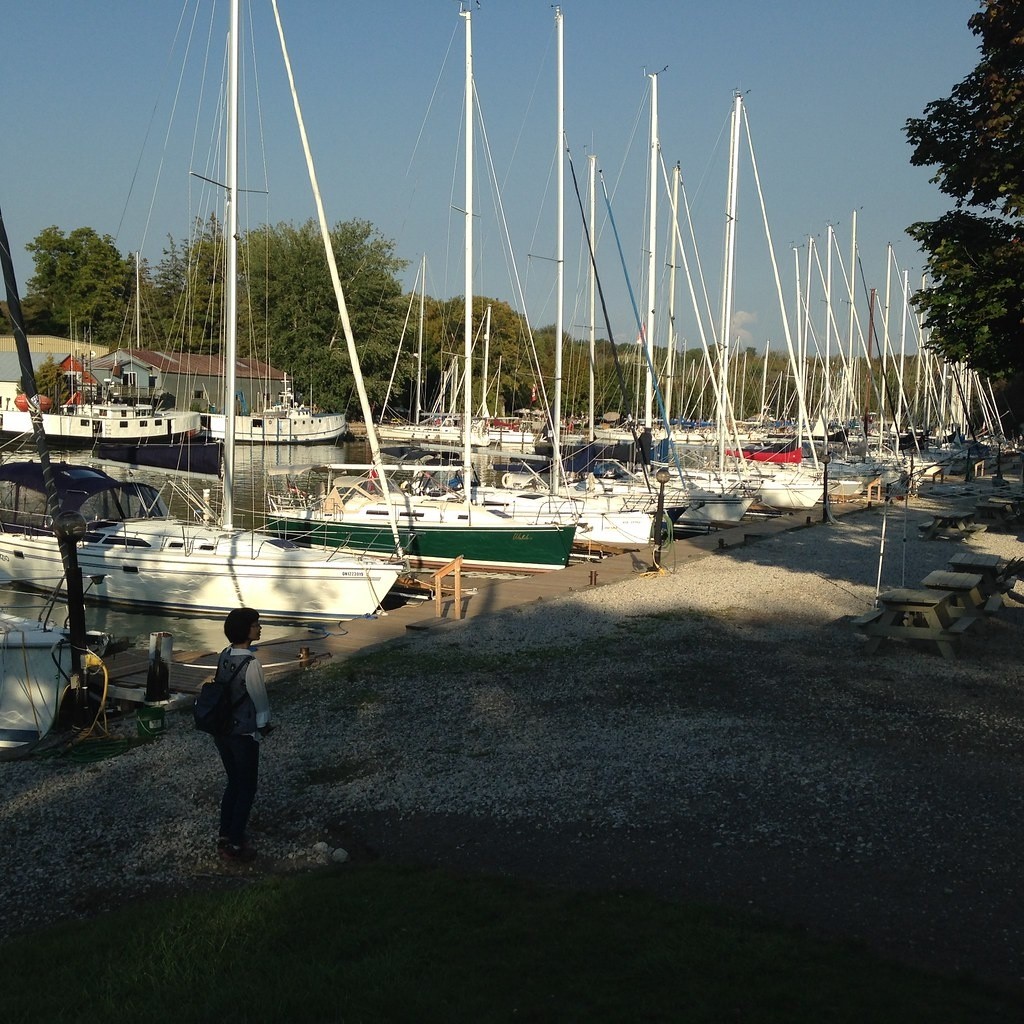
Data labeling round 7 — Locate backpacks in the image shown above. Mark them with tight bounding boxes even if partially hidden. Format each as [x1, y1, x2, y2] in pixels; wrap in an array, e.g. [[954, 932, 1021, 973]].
[[193, 646, 252, 736]]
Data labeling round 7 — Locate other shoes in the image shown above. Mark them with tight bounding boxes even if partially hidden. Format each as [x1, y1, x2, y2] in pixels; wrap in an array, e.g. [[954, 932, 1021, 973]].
[[216, 839, 258, 861]]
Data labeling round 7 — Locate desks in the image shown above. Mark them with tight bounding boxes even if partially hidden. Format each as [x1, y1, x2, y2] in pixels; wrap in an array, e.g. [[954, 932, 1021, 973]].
[[846, 487, 1023, 663]]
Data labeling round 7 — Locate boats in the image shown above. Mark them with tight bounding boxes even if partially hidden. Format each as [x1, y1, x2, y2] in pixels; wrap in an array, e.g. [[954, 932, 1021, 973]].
[[0, 1, 1015, 755]]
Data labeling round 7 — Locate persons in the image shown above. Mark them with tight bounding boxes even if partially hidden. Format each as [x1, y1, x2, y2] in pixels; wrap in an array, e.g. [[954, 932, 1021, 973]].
[[194, 607, 273, 862]]
